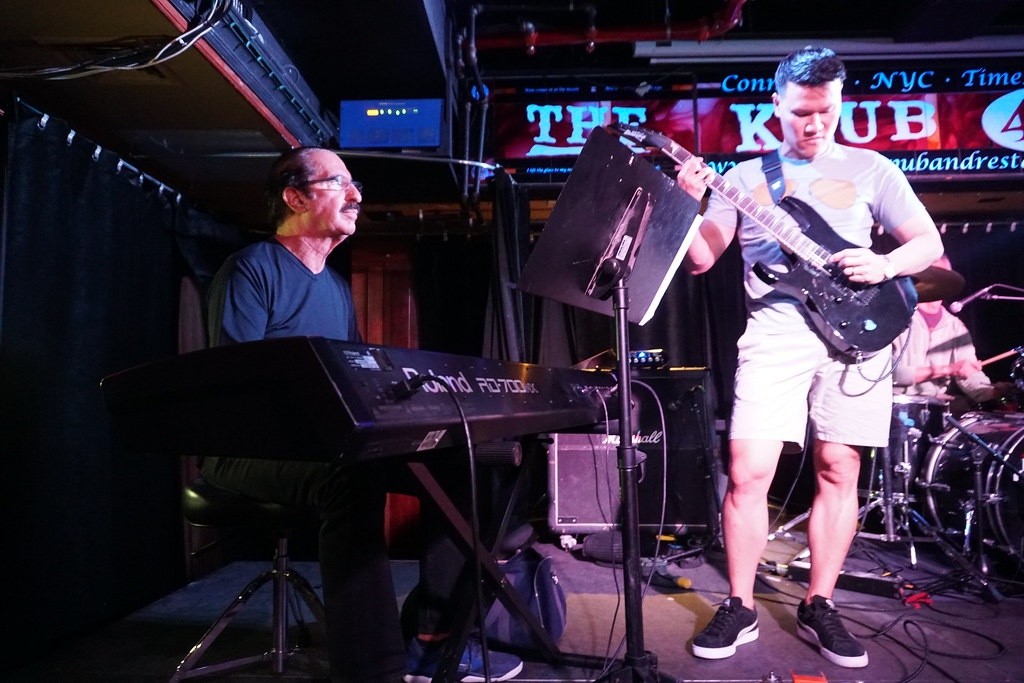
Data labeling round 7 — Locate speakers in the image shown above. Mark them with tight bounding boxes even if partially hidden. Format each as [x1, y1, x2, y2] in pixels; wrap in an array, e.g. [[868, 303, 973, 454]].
[[546, 370, 717, 538]]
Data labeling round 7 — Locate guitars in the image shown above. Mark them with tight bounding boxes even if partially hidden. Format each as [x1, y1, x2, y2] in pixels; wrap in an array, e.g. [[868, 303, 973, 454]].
[[606, 119, 919, 357]]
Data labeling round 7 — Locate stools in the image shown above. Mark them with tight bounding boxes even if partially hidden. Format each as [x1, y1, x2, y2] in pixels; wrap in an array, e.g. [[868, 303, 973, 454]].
[[168, 479, 325, 683]]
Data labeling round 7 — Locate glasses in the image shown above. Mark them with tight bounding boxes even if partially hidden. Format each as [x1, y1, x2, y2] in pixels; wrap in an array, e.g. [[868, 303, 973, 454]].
[[300, 174, 364, 194]]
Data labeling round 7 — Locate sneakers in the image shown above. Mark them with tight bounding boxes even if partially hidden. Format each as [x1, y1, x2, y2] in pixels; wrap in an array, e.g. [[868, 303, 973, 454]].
[[691, 597, 760, 660], [796, 594, 869, 668], [400, 632, 524, 683]]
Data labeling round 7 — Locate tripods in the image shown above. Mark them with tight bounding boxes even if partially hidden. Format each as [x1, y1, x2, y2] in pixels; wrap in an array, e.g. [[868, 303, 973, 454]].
[[663, 384, 776, 577], [768, 416, 1024, 605]]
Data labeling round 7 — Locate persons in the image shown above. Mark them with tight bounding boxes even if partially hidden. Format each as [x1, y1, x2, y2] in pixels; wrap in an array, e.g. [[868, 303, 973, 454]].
[[220, 146, 523, 683], [888, 253, 1011, 523], [676, 46, 943, 668]]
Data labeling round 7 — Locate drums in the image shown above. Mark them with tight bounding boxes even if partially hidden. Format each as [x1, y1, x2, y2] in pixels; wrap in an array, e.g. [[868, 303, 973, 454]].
[[924, 408, 1024, 564], [857, 393, 955, 503]]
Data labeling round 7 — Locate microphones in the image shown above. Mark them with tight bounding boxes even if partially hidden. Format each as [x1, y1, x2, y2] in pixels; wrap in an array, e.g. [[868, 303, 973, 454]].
[[948, 286, 995, 313], [666, 386, 695, 412]]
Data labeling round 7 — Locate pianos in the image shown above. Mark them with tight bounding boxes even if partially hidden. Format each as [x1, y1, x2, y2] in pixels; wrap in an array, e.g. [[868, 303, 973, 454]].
[[98, 334, 637, 683]]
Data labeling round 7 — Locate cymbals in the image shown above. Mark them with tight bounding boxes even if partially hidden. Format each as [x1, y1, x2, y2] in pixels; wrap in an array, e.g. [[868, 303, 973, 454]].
[[912, 265, 966, 304]]
